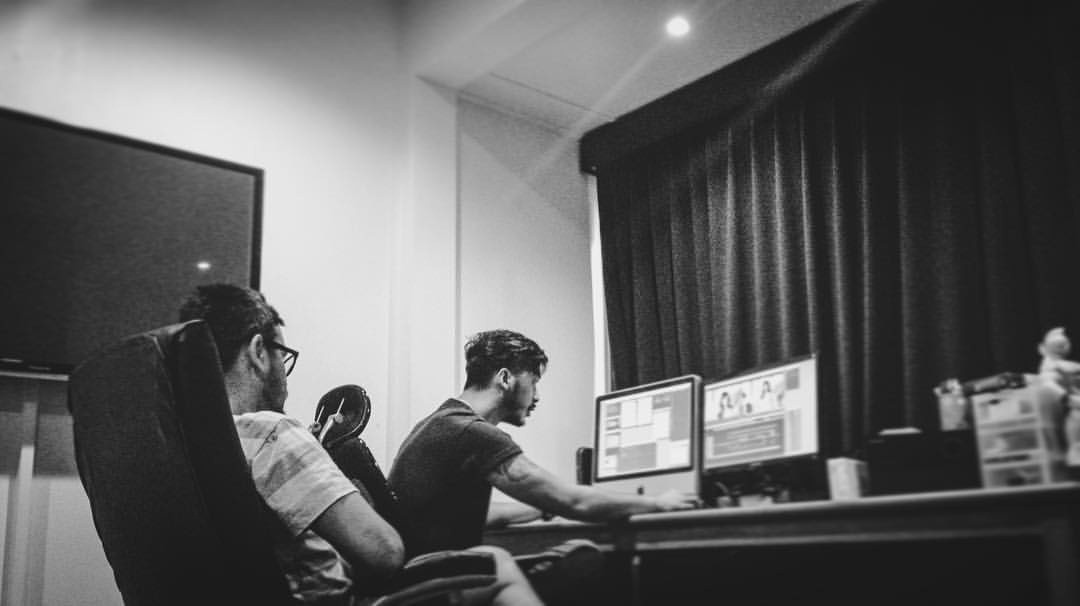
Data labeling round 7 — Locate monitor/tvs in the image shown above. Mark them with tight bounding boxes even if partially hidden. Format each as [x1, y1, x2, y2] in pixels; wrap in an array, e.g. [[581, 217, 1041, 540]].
[[590, 371, 703, 509], [701, 347, 828, 500]]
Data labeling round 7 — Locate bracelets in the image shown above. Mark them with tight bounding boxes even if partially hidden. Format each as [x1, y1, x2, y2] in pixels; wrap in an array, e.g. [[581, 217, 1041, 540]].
[[542, 510, 554, 521]]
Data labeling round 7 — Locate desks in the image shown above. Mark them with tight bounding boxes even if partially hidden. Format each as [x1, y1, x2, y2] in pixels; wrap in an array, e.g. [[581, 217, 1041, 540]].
[[472, 475, 1080, 606]]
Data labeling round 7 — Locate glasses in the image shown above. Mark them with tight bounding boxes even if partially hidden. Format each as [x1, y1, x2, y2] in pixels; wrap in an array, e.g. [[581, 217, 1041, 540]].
[[264, 341, 298, 376]]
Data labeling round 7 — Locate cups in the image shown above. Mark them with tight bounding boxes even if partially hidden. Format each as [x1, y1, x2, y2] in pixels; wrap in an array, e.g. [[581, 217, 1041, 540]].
[[826, 456, 861, 500]]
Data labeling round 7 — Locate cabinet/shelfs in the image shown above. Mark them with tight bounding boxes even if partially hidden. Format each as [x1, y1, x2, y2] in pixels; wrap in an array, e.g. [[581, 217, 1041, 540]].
[[969, 381, 1068, 490]]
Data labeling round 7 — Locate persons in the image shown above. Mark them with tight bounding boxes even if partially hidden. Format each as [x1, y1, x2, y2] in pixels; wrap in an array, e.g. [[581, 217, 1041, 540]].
[[178, 281, 406, 606], [382, 328, 706, 566]]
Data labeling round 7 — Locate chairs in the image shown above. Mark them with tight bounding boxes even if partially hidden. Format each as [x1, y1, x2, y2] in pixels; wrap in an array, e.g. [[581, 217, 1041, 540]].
[[308, 381, 607, 606], [63, 314, 499, 606]]
